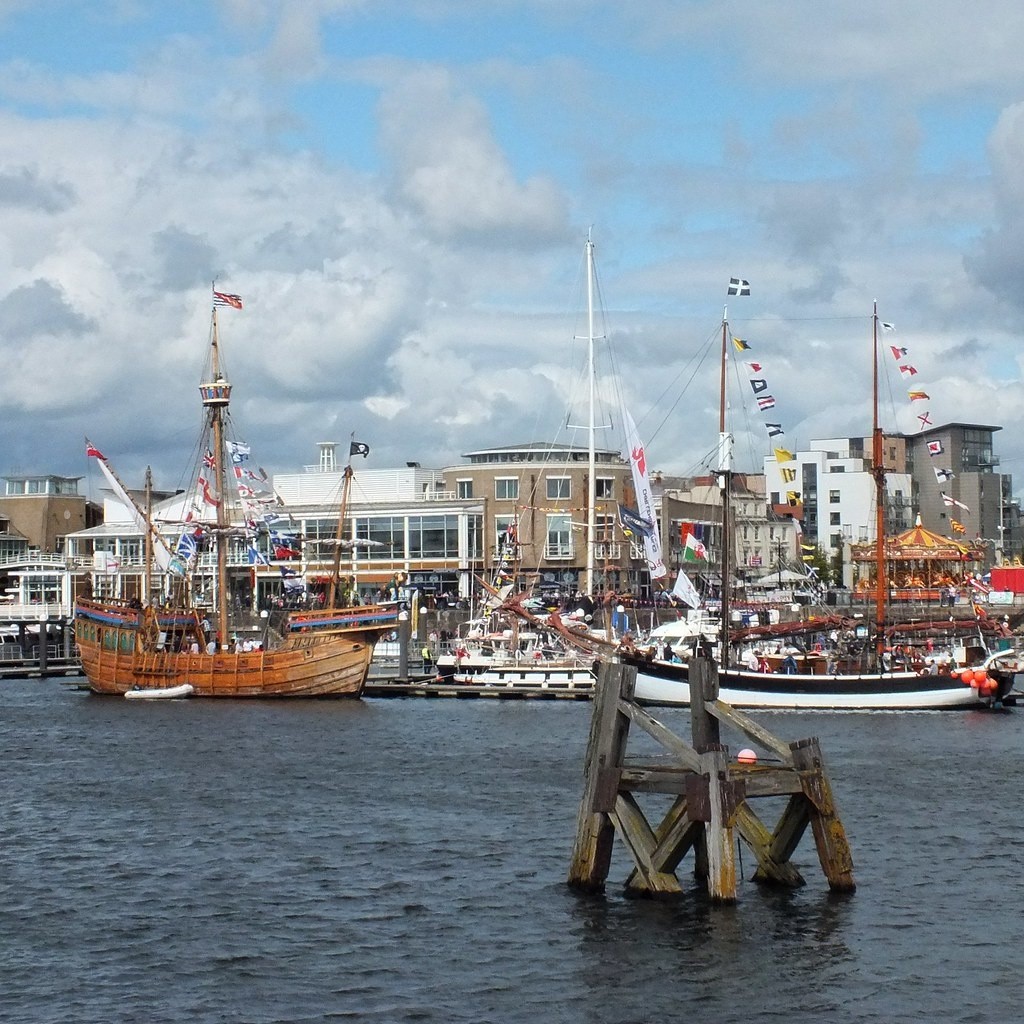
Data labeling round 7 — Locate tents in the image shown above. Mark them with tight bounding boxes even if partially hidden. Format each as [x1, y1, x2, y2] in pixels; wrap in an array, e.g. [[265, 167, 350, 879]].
[[760, 568, 808, 583]]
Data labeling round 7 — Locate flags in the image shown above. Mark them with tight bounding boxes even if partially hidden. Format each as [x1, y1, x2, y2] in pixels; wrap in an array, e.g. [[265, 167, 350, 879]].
[[214, 291, 243, 310], [88, 447, 103, 459], [225, 439, 268, 498], [241, 498, 266, 566], [683, 532, 707, 566], [732, 337, 776, 411], [618, 502, 655, 538], [727, 278, 751, 296], [879, 320, 990, 619], [765, 422, 802, 508], [264, 513, 301, 558], [197, 447, 222, 508], [482, 517, 518, 618], [278, 565, 304, 595], [106, 557, 119, 573], [170, 512, 203, 578], [673, 569, 702, 611]]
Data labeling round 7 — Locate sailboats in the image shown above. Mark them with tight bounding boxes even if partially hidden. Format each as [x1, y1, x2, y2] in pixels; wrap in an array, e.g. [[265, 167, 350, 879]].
[[423, 226, 1024, 717], [67, 277, 405, 702]]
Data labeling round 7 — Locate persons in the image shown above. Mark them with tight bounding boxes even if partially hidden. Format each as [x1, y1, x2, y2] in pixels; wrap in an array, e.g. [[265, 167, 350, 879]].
[[378, 628, 456, 674], [591, 656, 602, 678], [541, 642, 557, 660], [756, 627, 954, 675], [128, 592, 173, 614], [948, 584, 956, 607], [644, 637, 678, 664], [190, 617, 255, 656]]
